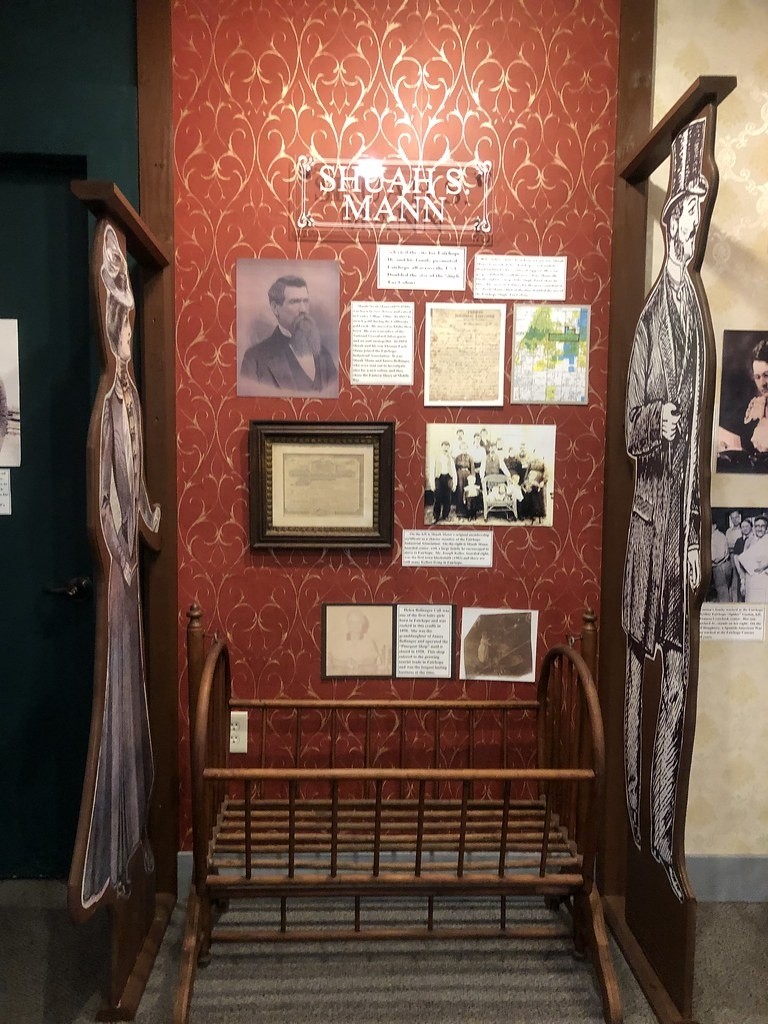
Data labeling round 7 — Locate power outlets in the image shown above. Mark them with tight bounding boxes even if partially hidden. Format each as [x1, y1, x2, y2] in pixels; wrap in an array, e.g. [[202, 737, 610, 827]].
[[229, 710, 248, 754]]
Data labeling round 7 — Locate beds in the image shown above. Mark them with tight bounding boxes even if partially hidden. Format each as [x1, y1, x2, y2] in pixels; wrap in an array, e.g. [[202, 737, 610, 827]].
[[172, 600, 625, 1024]]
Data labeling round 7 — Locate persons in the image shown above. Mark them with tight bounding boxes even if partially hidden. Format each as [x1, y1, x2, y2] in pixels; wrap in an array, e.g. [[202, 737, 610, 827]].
[[704, 509, 768, 603], [716, 339, 768, 474], [429, 429, 548, 525]]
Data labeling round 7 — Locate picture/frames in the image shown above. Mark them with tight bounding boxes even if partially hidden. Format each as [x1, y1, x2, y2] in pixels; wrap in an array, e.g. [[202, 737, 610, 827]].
[[321, 601, 395, 679], [511, 302, 589, 405], [424, 301, 505, 408], [247, 420, 397, 550]]
